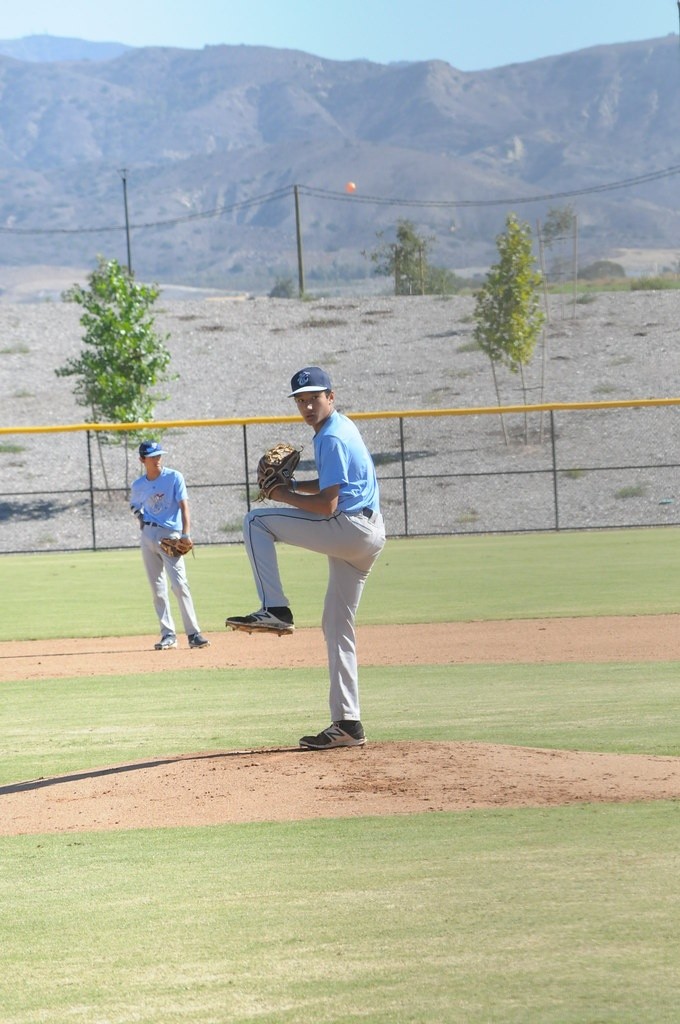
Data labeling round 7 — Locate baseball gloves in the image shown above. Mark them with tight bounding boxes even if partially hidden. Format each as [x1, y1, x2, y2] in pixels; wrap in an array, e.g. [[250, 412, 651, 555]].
[[159, 537, 196, 559], [251, 443, 305, 505]]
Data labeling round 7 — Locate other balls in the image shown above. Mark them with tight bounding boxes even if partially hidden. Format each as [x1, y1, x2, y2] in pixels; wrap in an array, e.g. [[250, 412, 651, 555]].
[[346, 182, 356, 192]]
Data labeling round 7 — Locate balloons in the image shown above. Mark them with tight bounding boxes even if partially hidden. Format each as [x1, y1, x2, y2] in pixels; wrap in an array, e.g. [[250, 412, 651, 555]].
[[346, 181, 355, 193]]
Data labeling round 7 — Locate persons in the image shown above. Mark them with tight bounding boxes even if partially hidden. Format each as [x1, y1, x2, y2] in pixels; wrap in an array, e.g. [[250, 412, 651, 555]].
[[225, 366, 386, 750], [129, 440, 210, 650]]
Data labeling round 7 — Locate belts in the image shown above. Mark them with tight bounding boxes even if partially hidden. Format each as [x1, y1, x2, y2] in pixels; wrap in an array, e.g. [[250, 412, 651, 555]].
[[144, 522, 164, 528]]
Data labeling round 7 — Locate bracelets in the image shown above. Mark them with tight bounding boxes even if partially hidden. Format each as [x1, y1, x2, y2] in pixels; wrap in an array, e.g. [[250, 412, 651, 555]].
[[181, 534, 190, 538], [292, 480, 297, 492]]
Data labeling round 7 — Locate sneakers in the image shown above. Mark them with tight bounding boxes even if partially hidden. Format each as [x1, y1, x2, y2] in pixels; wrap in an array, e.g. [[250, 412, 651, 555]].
[[225, 607, 295, 638], [188, 633, 210, 649], [299, 720, 367, 749], [154, 633, 177, 650]]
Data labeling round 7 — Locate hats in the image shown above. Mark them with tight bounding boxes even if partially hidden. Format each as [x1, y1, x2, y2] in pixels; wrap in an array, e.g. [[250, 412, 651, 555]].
[[139, 439, 168, 457], [286, 366, 332, 398]]
